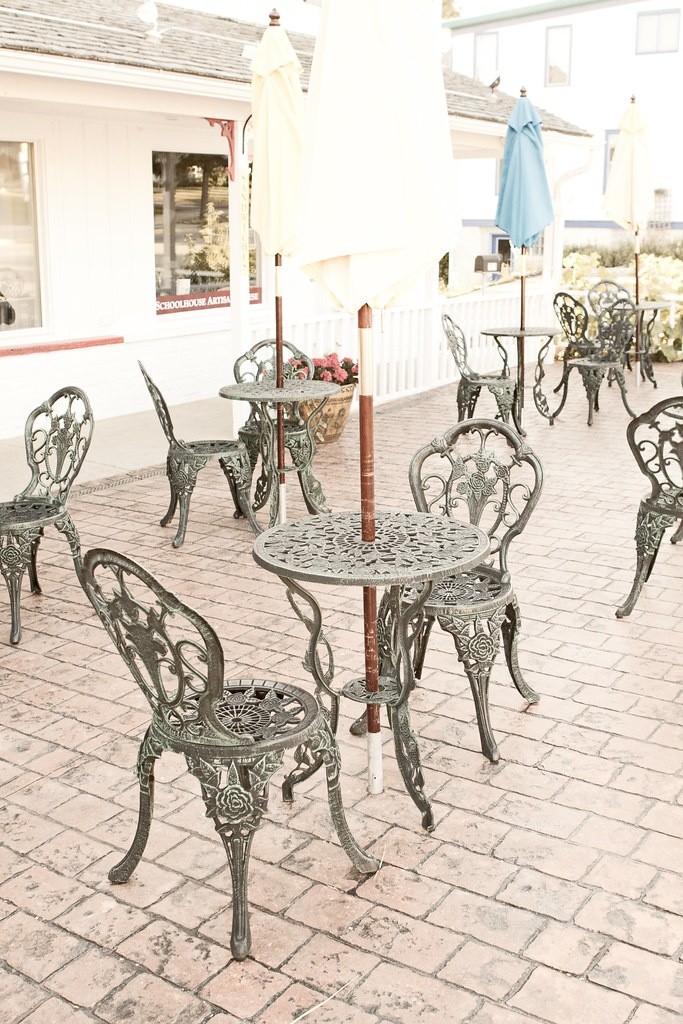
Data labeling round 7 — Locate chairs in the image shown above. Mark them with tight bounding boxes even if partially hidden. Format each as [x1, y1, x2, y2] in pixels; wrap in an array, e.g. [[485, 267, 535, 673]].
[[0, 386, 93, 645], [351, 419, 543, 765], [442, 314, 527, 447], [616, 397, 683, 619], [137, 360, 264, 548], [553, 279, 638, 426], [82, 548, 381, 960], [231, 339, 329, 518]]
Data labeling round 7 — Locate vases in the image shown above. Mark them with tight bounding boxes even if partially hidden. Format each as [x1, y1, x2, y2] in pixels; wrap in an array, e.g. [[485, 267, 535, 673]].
[[283, 384, 357, 443]]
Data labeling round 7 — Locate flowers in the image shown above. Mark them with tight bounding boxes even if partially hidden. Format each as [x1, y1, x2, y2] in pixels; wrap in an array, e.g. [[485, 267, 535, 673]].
[[265, 352, 359, 386]]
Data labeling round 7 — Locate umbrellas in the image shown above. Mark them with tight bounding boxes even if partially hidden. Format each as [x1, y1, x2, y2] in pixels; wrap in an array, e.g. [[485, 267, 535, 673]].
[[247, 4, 315, 524], [495, 86, 555, 408], [604, 91, 655, 386]]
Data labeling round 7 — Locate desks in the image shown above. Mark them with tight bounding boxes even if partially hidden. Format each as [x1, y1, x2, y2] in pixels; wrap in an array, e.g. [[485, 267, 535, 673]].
[[253, 509, 490, 832], [602, 302, 672, 388], [481, 327, 564, 425], [219, 380, 341, 516]]
